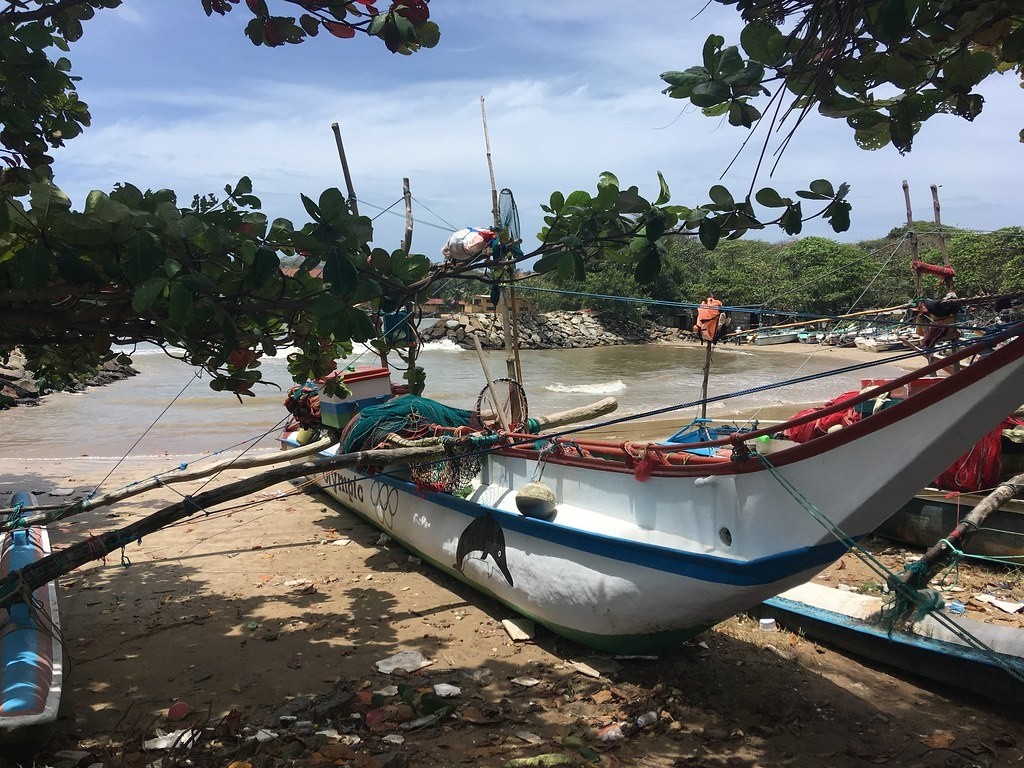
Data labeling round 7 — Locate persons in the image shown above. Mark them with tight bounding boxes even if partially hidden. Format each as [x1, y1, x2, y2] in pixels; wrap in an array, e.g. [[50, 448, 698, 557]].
[[735, 326, 742, 346]]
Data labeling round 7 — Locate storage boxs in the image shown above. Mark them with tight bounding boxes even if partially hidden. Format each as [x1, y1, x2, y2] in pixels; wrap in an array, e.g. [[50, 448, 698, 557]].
[[318, 365, 392, 429]]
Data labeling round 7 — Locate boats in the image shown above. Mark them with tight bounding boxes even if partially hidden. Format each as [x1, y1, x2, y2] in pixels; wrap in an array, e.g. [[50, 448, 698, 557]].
[[277, 298, 1024, 712], [0, 487, 64, 734]]
